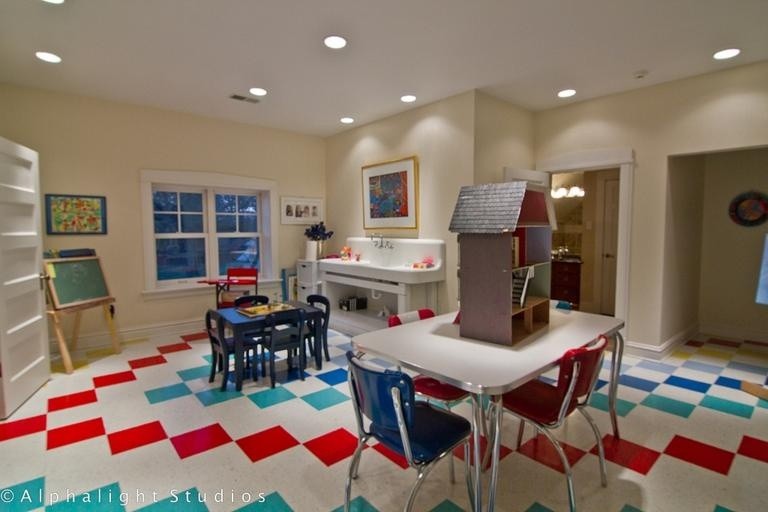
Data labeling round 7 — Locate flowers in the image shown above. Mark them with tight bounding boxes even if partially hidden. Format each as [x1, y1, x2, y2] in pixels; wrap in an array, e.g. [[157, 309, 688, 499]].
[[304, 222, 334, 244]]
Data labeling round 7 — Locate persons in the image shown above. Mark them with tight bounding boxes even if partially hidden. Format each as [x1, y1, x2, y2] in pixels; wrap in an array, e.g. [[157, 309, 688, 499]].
[[405, 255, 436, 270], [295, 205, 303, 216], [304, 206, 309, 216], [286, 205, 293, 216], [311, 206, 318, 216]]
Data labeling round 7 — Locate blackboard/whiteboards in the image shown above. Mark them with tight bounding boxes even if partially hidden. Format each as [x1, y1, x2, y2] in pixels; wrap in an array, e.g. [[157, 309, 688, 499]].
[[48, 258, 110, 306]]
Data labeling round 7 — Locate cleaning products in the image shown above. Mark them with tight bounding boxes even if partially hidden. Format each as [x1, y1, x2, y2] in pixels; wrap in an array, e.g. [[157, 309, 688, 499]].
[[340, 247, 352, 260]]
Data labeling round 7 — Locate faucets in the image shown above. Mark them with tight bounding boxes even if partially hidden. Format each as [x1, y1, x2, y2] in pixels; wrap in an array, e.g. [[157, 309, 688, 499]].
[[377, 233, 395, 251], [557, 247, 563, 260], [369, 233, 377, 248], [565, 243, 569, 253]]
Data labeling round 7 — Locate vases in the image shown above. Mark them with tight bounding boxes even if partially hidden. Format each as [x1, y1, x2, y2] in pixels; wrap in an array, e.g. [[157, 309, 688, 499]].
[[306, 241, 322, 259]]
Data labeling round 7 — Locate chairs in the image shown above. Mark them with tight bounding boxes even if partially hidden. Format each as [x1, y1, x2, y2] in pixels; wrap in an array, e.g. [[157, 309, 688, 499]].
[[197, 269, 257, 309], [234, 295, 278, 368], [206, 309, 258, 391], [345, 350, 475, 512], [481, 334, 609, 512], [258, 307, 306, 388], [389, 309, 488, 485], [298, 295, 330, 361]]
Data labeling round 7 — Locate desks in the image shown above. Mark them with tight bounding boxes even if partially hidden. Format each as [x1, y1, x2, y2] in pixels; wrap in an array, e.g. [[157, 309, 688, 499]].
[[217, 301, 322, 391], [352, 311, 626, 512]]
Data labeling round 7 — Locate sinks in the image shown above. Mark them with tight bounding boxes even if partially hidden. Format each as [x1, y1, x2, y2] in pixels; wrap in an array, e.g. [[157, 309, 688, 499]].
[[558, 257, 584, 264], [361, 261, 403, 270]]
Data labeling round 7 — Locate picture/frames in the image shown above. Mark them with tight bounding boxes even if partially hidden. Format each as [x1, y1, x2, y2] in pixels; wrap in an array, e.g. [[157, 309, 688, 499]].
[[361, 155, 418, 230], [281, 197, 323, 225]]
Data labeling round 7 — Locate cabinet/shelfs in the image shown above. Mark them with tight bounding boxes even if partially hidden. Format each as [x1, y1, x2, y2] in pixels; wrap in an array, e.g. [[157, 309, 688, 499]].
[[295, 259, 322, 305]]
